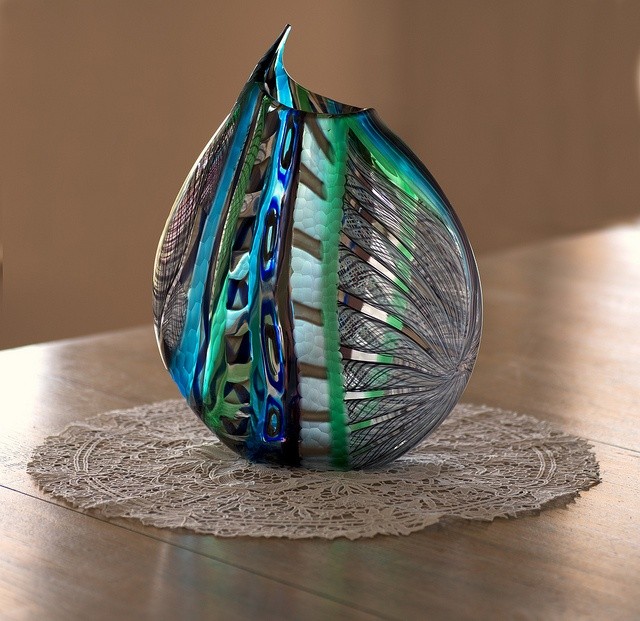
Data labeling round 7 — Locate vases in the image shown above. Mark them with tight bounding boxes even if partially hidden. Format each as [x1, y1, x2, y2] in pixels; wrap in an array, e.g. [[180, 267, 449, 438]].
[[151, 18, 484, 470]]
